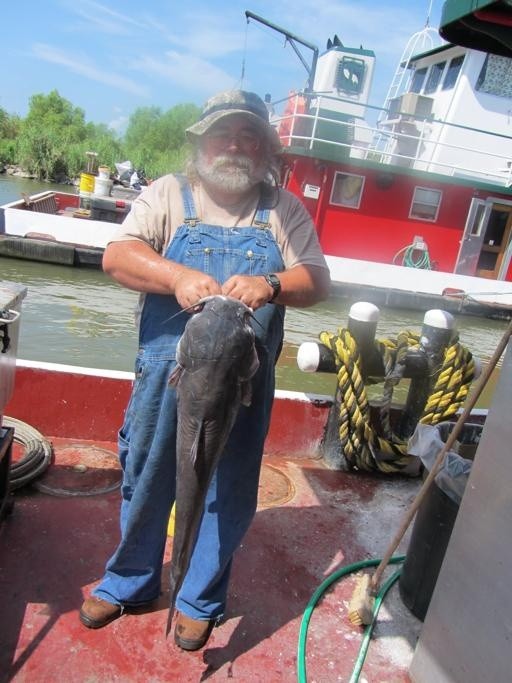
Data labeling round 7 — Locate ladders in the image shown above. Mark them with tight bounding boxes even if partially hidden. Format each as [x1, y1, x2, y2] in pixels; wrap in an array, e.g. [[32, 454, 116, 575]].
[[23, 193, 59, 215]]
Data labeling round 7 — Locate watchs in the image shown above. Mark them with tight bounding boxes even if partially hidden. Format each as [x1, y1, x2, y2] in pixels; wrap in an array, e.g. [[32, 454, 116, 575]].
[[262, 271, 281, 305]]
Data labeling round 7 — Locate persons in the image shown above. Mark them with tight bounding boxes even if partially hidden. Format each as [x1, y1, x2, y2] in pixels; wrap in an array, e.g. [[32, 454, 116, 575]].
[[78, 90, 332, 651]]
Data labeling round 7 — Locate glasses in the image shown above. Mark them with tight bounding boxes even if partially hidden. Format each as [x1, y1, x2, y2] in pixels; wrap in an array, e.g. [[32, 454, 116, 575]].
[[208, 130, 259, 150]]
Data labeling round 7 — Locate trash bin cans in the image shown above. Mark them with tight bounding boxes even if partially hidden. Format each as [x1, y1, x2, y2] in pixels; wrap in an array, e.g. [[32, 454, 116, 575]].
[[398, 421, 483, 623]]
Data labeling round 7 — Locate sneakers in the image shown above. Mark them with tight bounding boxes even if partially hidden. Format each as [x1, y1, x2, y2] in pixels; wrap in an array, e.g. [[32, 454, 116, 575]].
[[174, 611, 212, 651], [79, 595, 122, 628]]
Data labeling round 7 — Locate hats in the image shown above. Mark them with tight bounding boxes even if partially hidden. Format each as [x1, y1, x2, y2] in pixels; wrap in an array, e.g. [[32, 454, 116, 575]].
[[186, 89, 281, 153]]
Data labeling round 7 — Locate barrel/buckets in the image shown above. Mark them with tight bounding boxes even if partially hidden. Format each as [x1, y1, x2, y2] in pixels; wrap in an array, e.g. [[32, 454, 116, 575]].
[[98, 167, 111, 179], [79, 171, 97, 192], [94, 176, 114, 196]]
